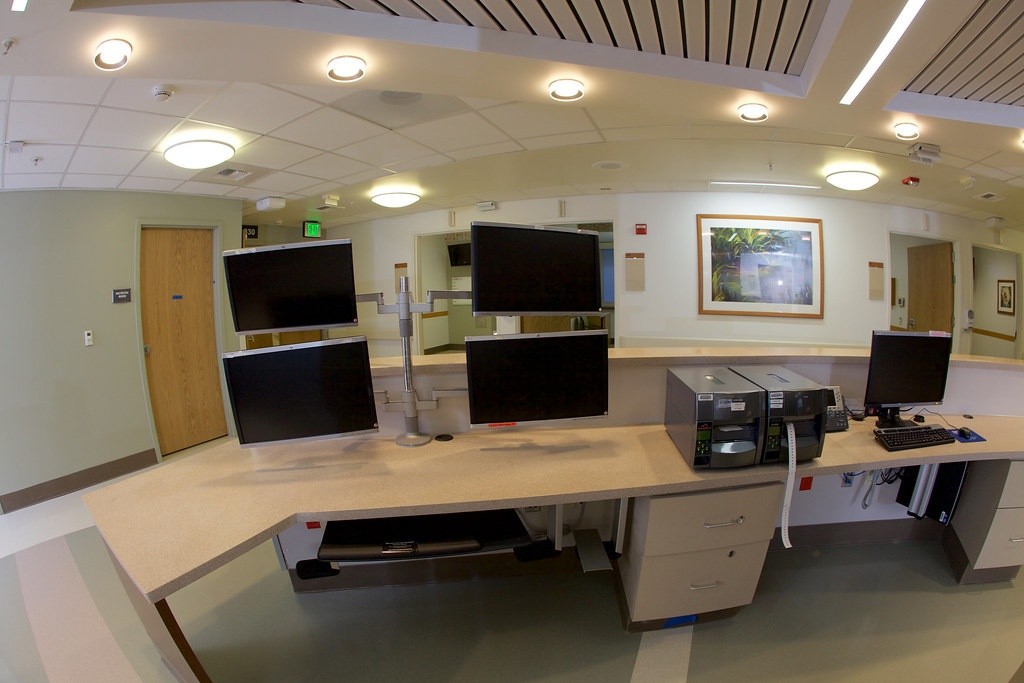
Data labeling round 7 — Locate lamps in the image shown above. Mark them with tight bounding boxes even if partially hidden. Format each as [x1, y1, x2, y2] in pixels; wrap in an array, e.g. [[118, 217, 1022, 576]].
[[93, 40, 132, 72], [738, 104, 768, 122], [825, 171, 880, 190], [326, 56, 366, 83], [549, 79, 585, 102], [371, 193, 421, 208], [164, 140, 235, 168], [894, 123, 920, 141]]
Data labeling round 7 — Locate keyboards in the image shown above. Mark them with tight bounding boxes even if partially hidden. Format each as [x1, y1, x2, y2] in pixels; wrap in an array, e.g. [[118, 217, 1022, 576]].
[[873, 424, 956, 452]]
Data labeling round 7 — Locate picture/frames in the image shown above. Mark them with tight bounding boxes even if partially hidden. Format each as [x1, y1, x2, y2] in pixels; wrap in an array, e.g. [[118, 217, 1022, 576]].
[[696, 214, 824, 319], [997, 279, 1015, 315]]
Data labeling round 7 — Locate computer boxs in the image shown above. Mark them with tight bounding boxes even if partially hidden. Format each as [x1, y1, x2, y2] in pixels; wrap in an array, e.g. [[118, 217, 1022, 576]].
[[896, 461, 970, 526]]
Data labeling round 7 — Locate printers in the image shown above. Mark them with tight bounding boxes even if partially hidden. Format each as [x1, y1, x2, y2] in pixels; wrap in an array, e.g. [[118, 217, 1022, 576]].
[[664, 367, 767, 469], [729, 365, 829, 464]]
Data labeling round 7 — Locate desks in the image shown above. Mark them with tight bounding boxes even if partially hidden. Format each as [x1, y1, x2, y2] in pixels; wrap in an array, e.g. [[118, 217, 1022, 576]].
[[80, 413, 1024, 683]]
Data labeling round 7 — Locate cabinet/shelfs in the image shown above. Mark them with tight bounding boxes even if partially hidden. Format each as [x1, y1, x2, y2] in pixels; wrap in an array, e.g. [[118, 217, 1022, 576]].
[[942, 460, 1024, 585], [616, 482, 785, 632]]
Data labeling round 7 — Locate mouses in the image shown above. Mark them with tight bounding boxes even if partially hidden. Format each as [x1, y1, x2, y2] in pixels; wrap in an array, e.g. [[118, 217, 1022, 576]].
[[958, 427, 970, 439]]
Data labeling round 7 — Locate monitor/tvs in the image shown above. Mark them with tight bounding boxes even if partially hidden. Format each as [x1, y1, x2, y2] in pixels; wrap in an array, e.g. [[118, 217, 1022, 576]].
[[470, 221, 603, 317], [863, 330, 952, 428], [465, 329, 610, 430], [222, 335, 379, 448], [223, 238, 357, 336], [448, 243, 472, 266]]
